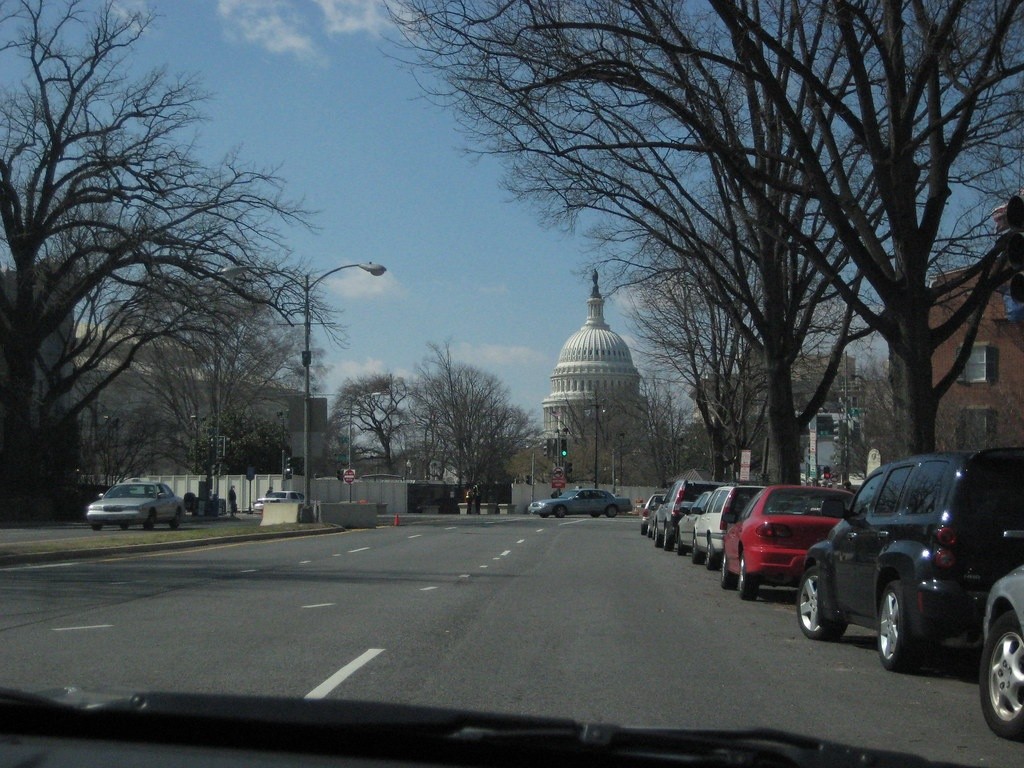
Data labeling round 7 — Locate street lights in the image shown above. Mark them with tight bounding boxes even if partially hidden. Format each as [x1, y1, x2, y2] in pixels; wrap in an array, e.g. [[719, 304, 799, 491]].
[[221, 261, 390, 523], [394, 514, 402, 526], [585, 390, 607, 489], [348, 391, 383, 504]]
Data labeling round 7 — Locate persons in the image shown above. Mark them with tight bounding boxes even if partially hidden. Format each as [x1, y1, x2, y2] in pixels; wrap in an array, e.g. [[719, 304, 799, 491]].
[[465, 488, 481, 516], [228, 485, 238, 518], [267, 487, 273, 496], [551, 488, 563, 498]]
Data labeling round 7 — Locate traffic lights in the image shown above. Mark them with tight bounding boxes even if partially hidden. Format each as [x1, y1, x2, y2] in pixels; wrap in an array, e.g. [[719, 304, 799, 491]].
[[850, 418, 871, 449], [283, 455, 292, 476], [543, 437, 554, 459], [565, 462, 574, 476], [834, 418, 843, 444], [1005, 192, 1024, 308], [561, 439, 570, 458], [218, 436, 226, 458], [224, 435, 234, 459], [824, 467, 832, 479]]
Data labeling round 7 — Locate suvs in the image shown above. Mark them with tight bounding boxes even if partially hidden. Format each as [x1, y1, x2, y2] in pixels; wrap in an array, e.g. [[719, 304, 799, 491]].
[[793, 445, 1024, 674], [654, 478, 741, 551]]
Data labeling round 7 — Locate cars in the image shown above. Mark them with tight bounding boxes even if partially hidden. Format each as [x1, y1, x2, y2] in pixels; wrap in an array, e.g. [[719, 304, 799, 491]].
[[980, 562, 1024, 741], [86, 477, 186, 531], [529, 487, 634, 519], [252, 490, 314, 515], [721, 485, 855, 600], [640, 493, 669, 536], [691, 485, 788, 571], [675, 490, 716, 558]]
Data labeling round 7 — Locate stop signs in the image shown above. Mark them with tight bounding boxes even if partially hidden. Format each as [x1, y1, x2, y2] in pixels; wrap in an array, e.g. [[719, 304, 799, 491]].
[[343, 469, 356, 484]]
